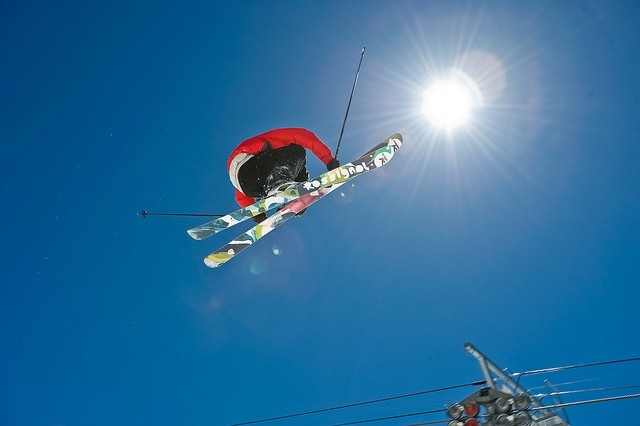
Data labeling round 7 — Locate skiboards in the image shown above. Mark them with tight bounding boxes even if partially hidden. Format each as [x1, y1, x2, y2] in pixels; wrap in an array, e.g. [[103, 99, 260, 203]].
[[187, 133, 402, 269]]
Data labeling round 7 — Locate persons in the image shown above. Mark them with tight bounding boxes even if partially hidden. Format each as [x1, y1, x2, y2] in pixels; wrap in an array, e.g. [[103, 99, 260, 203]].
[[228, 127, 339, 224]]
